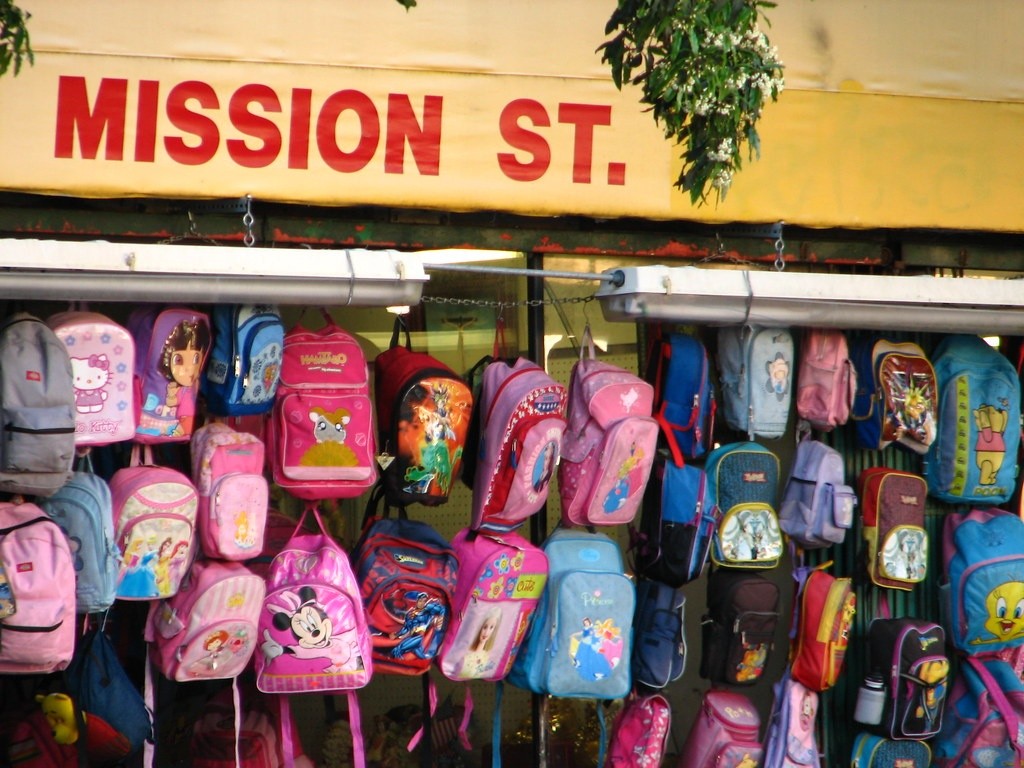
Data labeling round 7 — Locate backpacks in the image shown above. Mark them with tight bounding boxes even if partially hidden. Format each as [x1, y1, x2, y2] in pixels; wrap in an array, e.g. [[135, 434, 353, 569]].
[[549, 324, 660, 529], [373, 313, 475, 509], [847, 330, 938, 457], [465, 352, 574, 537], [643, 324, 725, 468], [714, 323, 795, 446], [1, 312, 145, 768], [610, 431, 960, 768], [145, 560, 313, 768], [188, 415, 272, 567], [280, 304, 384, 502], [259, 503, 488, 768], [410, 526, 550, 768], [931, 653, 1024, 768], [941, 504, 1024, 664], [107, 441, 200, 607], [792, 326, 860, 435], [130, 305, 209, 446], [485, 526, 636, 766], [201, 305, 284, 416], [920, 332, 1022, 512]]
